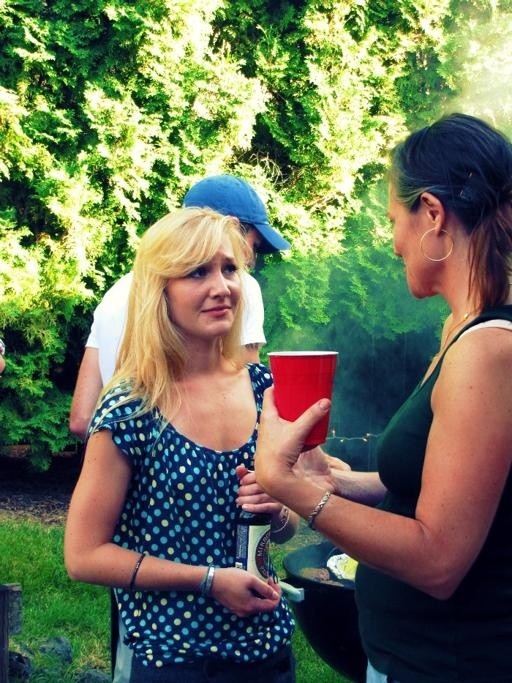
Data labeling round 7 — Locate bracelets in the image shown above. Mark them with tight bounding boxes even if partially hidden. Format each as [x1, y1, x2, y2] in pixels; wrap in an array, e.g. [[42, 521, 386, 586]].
[[130, 553, 146, 589], [308, 491, 331, 529], [271, 513, 290, 532], [203, 566, 214, 596]]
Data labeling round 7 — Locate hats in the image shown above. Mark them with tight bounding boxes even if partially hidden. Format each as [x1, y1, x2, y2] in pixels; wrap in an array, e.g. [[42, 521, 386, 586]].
[[184, 173, 292, 251]]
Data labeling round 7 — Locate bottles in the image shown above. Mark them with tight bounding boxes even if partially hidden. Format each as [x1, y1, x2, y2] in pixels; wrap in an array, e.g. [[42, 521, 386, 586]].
[[236, 458, 270, 582]]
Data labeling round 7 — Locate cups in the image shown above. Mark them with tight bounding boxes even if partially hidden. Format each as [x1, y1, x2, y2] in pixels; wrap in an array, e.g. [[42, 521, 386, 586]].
[[269, 350, 337, 446]]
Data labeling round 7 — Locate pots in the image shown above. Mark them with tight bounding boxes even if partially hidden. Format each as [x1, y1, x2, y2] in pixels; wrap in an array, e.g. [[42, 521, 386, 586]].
[[275, 541, 364, 682]]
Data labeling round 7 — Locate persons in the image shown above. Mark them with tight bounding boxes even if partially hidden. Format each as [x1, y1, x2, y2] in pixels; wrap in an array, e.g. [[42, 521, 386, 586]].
[[253, 113, 512, 683]]
